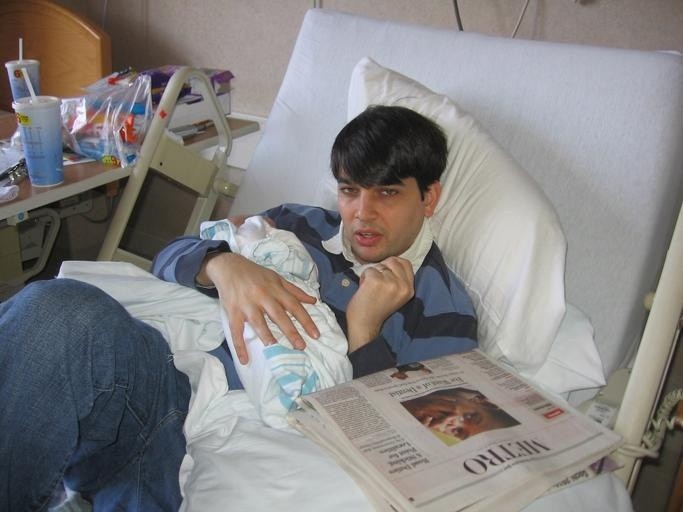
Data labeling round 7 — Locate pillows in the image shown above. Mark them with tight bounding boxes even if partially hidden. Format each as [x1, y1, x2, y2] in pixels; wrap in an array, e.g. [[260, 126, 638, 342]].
[[340, 54, 568, 380]]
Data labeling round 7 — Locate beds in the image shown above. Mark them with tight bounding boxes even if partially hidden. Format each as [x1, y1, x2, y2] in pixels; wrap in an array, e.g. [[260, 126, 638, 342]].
[[149, 8, 683, 512]]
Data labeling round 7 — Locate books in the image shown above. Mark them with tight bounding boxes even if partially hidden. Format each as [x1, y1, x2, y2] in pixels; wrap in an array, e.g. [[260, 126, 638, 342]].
[[166, 115, 227, 140], [62, 151, 100, 168]]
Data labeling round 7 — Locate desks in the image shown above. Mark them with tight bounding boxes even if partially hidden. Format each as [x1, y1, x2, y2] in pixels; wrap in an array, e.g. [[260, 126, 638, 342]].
[[0, 100, 260, 300]]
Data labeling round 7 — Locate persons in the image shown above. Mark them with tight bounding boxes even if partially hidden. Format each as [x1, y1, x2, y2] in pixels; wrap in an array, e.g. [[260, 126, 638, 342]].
[[402, 384, 519, 444], [398, 362, 432, 374], [1, 103, 481, 512], [384, 367, 408, 381]]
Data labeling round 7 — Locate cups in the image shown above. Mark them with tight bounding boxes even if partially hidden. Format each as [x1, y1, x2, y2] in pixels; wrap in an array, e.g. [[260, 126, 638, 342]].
[[11, 95, 65, 188], [5, 60, 41, 101]]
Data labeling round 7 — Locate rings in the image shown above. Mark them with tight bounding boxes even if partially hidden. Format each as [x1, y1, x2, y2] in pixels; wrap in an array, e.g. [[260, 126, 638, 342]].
[[378, 267, 392, 274]]
